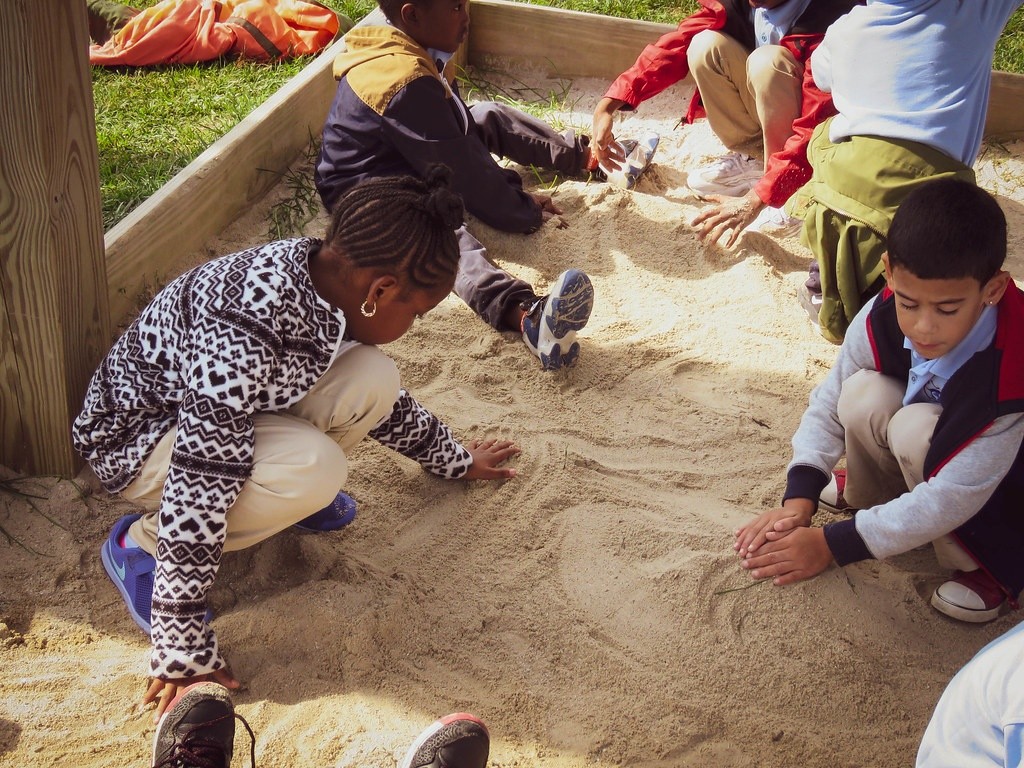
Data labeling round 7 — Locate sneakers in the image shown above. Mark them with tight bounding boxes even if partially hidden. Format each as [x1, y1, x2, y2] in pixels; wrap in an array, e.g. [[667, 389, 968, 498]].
[[293, 490, 357, 533], [819, 467, 860, 513], [519, 269, 594, 373], [399, 711, 490, 768], [101, 513, 216, 637], [150, 681, 235, 768], [687, 152, 764, 200], [784, 271, 844, 345], [930, 568, 1008, 623], [596, 132, 660, 189], [745, 205, 803, 238]]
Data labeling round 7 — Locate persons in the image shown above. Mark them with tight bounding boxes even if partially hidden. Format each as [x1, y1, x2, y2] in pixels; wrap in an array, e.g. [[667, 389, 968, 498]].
[[313, 0, 659, 372], [733, 178, 1024, 624], [150, 680, 491, 768], [71, 163, 521, 725], [913, 619, 1024, 768], [806, 0, 1021, 347], [588, 0, 866, 252]]
